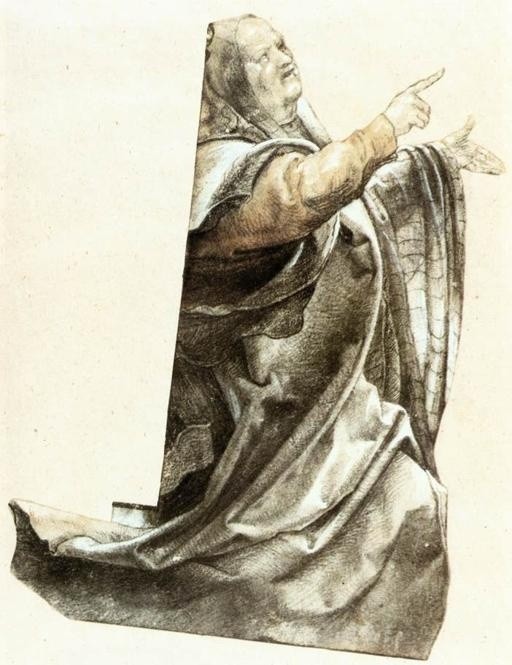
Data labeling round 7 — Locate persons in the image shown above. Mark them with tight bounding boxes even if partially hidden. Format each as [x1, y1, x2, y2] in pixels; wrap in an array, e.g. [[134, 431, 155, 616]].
[[8, 12, 506, 662]]
